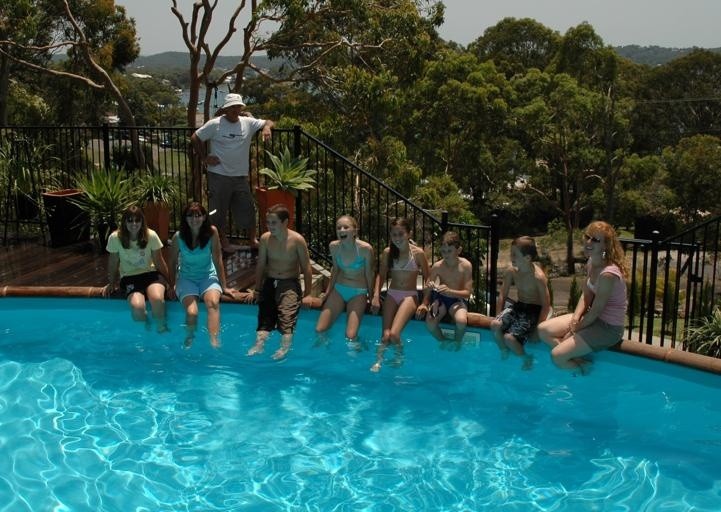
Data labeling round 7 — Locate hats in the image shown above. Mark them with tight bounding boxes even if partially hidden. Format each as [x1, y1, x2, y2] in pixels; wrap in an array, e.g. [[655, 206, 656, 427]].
[[221, 93, 247, 110]]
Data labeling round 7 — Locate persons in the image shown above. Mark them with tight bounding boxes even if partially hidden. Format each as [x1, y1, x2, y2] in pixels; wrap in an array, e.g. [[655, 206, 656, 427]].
[[426, 231, 473, 352], [101, 205, 173, 333], [537, 220, 630, 377], [490, 235, 551, 371], [191, 93, 275, 258], [369, 216, 431, 373], [243, 203, 314, 360], [315, 214, 375, 353], [168, 201, 240, 348]]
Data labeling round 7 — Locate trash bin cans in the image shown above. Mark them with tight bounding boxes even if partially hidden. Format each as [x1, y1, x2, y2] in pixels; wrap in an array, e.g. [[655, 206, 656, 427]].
[[41, 189, 89, 248]]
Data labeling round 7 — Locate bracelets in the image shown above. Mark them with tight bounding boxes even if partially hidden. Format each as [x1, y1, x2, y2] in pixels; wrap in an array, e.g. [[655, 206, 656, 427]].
[[253, 289, 261, 294]]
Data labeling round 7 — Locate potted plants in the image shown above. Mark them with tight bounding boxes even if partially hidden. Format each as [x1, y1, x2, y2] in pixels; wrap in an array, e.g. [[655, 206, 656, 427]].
[[256, 147, 319, 230], [2, 133, 182, 249]]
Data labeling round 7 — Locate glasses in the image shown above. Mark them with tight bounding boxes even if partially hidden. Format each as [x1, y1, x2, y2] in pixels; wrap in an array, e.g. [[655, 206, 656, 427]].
[[126, 216, 142, 224], [583, 233, 601, 244]]
